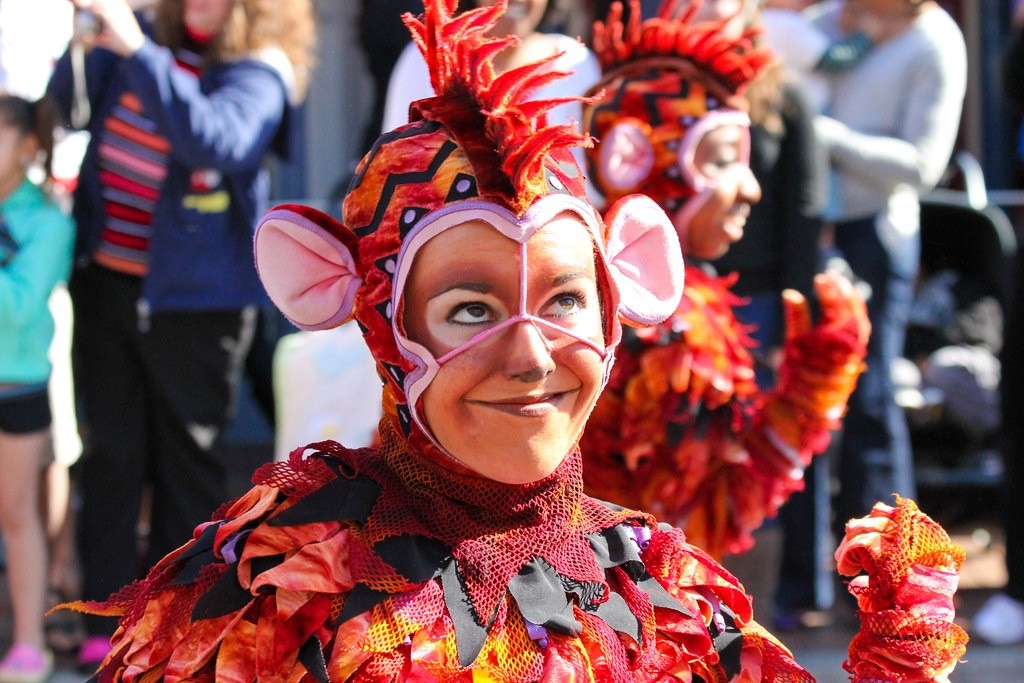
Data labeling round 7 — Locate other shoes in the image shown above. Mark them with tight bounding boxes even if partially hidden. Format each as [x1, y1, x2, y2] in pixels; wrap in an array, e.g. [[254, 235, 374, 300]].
[[0, 644, 54, 682], [79, 637, 113, 669]]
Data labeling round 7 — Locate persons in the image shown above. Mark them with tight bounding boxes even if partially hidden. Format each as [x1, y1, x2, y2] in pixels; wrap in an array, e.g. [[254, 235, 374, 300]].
[[578, 0, 871, 561], [758, 0, 887, 268], [974, 0, 1024, 648], [46, 1, 319, 669], [40, 0, 972, 683], [0, 92, 75, 683], [379, 0, 608, 209], [768, 0, 969, 627], [672, 0, 826, 401]]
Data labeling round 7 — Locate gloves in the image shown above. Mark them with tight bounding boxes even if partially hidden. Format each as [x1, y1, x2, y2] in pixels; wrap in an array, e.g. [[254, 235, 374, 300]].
[[832, 495, 967, 642], [762, 261, 871, 456]]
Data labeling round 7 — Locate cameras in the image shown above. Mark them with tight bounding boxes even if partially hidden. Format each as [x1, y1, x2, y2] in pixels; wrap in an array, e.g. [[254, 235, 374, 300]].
[[74, 7, 103, 37]]
